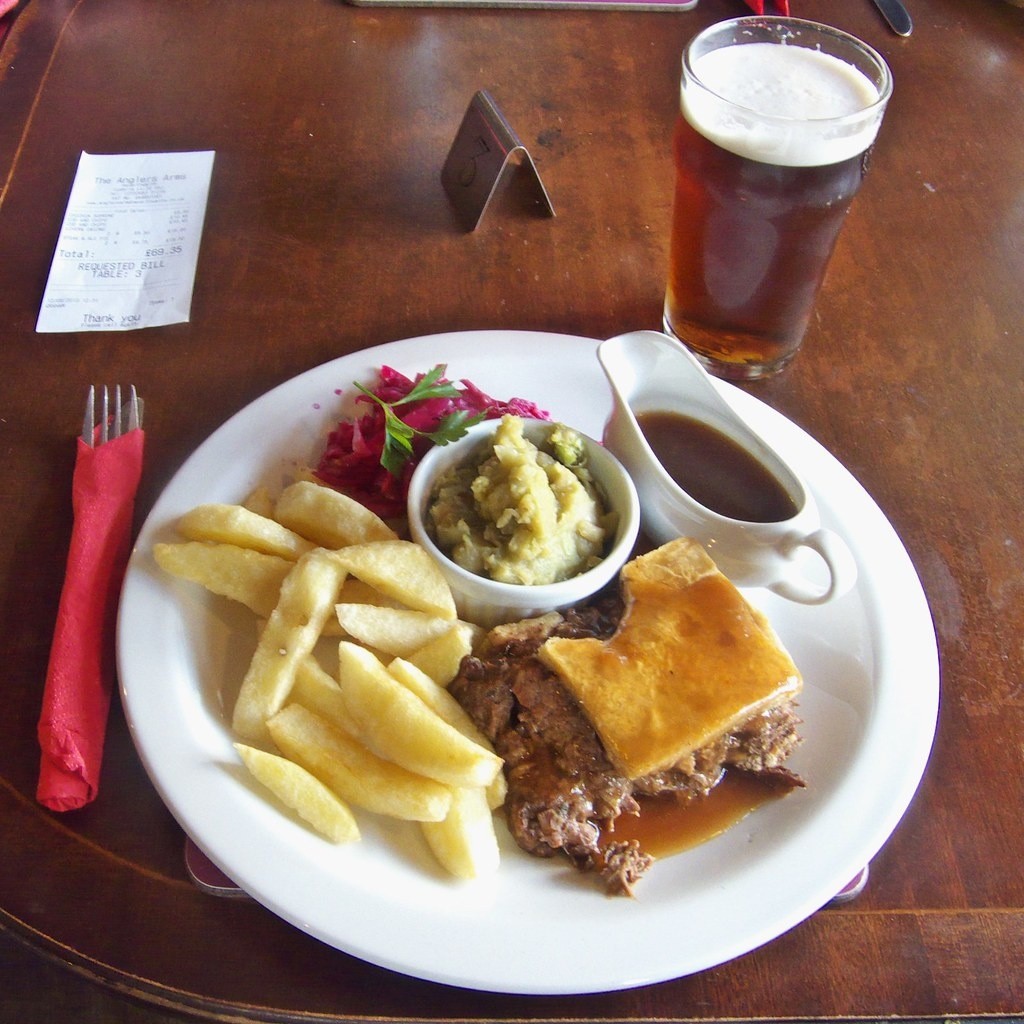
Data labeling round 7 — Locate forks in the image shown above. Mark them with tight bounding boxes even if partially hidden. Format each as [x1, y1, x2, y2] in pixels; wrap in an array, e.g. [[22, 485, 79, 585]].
[[36, 384, 140, 811]]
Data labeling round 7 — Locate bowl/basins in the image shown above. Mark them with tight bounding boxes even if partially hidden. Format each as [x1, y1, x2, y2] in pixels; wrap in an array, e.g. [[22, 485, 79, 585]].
[[406, 417, 641, 630]]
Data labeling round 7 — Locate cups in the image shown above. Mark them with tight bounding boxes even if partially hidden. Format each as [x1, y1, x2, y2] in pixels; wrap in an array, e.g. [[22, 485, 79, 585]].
[[596, 330, 858, 606], [661, 15, 894, 380]]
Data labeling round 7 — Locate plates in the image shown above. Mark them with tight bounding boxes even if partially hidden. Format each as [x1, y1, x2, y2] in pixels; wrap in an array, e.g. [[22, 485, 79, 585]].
[[115, 331, 940, 995]]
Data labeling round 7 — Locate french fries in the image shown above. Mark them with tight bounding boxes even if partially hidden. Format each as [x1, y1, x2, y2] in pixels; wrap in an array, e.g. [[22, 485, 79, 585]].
[[153, 481, 507, 881]]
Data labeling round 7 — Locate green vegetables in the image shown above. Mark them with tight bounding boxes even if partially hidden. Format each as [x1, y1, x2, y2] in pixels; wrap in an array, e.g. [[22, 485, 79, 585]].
[[352, 367, 487, 475]]
